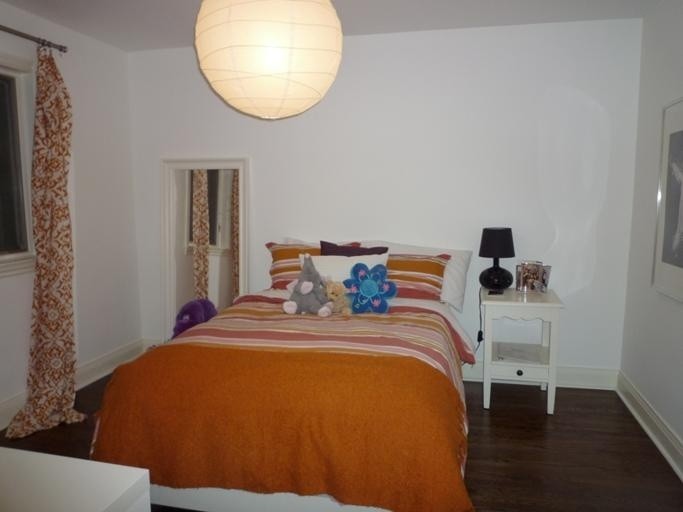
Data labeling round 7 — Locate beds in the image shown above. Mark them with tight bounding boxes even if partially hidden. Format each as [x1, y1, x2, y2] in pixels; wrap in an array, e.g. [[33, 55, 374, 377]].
[[91, 287, 476, 512]]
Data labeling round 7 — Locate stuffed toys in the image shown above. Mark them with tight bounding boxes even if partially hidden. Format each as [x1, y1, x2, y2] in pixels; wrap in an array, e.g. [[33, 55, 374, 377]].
[[324, 282, 352, 315], [198, 299, 218, 321], [172, 301, 205, 339], [283, 253, 334, 317]]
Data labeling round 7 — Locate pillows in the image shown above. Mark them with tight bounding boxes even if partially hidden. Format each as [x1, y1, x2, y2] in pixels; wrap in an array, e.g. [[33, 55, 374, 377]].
[[252, 237, 475, 316]]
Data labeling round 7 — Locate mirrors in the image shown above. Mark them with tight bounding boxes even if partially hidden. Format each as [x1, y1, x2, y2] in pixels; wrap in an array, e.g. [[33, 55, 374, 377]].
[[162, 158, 245, 339]]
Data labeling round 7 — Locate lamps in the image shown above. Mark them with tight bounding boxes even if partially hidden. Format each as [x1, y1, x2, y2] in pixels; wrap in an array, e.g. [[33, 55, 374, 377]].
[[192, 0, 345, 123], [475, 226, 516, 296]]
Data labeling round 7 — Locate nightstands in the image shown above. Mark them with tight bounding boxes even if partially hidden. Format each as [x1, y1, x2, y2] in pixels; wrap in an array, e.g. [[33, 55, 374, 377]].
[[476, 286, 566, 416]]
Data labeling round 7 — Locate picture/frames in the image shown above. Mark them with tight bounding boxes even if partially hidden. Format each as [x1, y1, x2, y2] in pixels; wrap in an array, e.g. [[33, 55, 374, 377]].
[[649, 97, 683, 305]]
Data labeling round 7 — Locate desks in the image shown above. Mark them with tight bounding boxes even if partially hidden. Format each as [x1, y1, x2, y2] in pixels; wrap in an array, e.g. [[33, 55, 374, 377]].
[[0, 439, 152, 511]]
